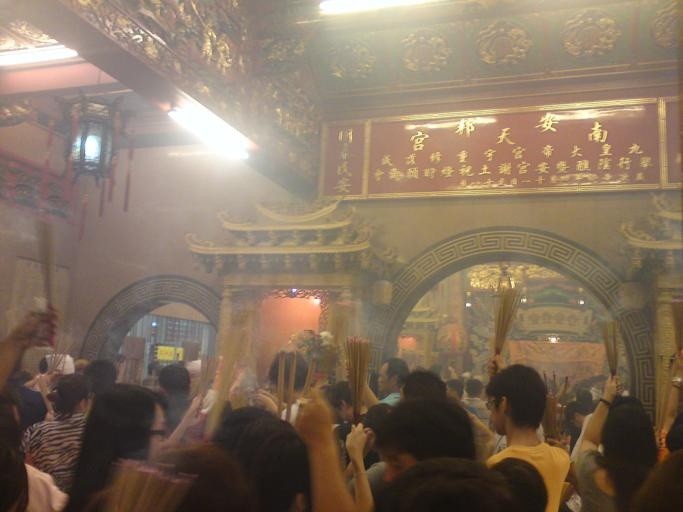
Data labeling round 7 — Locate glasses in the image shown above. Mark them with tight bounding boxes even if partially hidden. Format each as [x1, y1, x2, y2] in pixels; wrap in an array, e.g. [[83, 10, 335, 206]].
[[150, 421, 167, 441]]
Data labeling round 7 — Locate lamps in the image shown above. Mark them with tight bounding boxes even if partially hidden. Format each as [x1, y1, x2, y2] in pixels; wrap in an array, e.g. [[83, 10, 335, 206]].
[[48, 65, 138, 189]]
